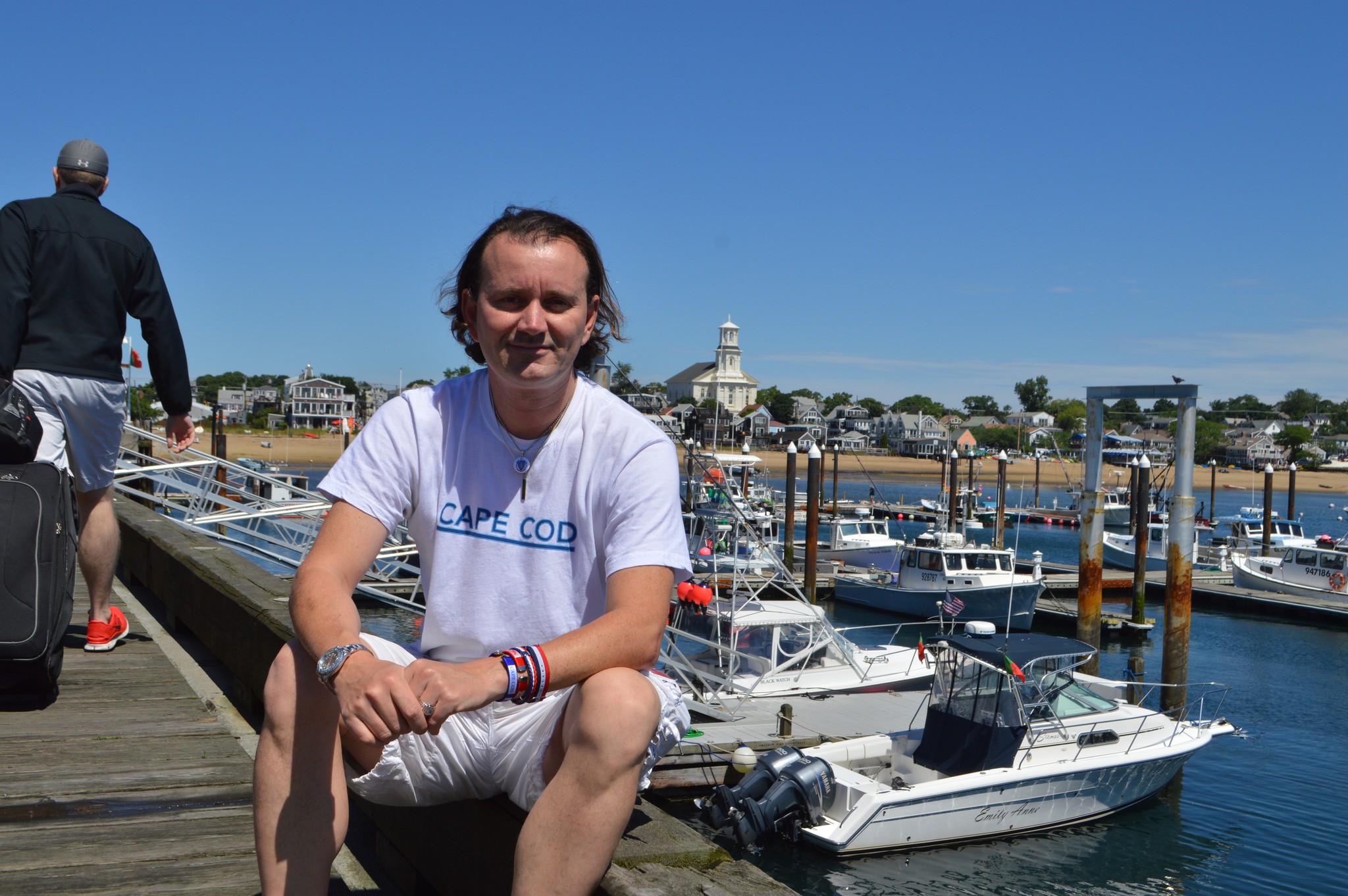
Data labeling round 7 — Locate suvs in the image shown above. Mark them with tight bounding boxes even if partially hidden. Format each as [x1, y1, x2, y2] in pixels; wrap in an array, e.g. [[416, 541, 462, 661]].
[[1005, 448, 1018, 457]]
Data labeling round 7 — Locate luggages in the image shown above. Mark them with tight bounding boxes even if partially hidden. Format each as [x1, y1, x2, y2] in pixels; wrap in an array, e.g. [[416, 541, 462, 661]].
[[0, 462, 78, 700]]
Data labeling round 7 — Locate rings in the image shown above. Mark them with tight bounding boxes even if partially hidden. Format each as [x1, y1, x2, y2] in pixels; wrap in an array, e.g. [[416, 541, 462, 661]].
[[417, 698, 435, 716]]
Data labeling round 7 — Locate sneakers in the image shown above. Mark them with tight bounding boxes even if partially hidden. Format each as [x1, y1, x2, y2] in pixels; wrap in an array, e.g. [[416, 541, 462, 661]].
[[82, 605, 130, 653]]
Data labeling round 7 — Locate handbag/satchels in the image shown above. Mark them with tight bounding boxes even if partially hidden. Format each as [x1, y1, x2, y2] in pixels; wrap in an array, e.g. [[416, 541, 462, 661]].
[[0, 378, 43, 463]]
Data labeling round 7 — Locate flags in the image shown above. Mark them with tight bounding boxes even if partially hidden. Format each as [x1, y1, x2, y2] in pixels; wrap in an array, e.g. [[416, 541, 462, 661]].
[[1004, 655, 1025, 684], [120, 336, 142, 368], [918, 635, 924, 664], [942, 590, 966, 617]]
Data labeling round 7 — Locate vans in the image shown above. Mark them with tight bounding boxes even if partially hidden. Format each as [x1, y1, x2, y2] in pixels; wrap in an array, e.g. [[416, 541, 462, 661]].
[[986, 449, 995, 453]]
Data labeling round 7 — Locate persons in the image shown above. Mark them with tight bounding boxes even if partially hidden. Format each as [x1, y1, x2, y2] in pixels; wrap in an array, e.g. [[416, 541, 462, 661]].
[[253, 204, 694, 896], [0, 138, 192, 650]]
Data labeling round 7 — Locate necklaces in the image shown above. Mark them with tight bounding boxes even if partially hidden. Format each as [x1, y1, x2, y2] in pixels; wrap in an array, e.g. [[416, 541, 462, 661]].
[[488, 379, 576, 500]]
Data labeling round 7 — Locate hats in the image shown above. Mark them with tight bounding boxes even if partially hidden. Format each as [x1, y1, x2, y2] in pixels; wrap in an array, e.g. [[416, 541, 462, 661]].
[[55, 138, 108, 176]]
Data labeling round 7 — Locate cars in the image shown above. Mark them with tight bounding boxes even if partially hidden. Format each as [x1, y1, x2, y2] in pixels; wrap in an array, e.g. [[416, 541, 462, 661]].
[[1328, 454, 1338, 460]]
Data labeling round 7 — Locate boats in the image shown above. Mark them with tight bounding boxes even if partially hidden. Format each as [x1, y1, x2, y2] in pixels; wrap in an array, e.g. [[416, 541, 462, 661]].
[[1057, 451, 1348, 606], [234, 454, 333, 521], [622, 462, 1240, 859]]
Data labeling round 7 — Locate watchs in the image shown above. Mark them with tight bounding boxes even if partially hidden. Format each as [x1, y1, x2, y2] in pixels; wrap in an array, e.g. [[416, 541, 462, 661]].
[[316, 644, 373, 697]]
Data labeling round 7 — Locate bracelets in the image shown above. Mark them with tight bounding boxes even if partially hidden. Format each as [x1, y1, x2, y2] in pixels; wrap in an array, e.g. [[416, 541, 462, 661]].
[[501, 649, 528, 700], [508, 647, 537, 705], [521, 644, 550, 703], [488, 650, 518, 702]]
